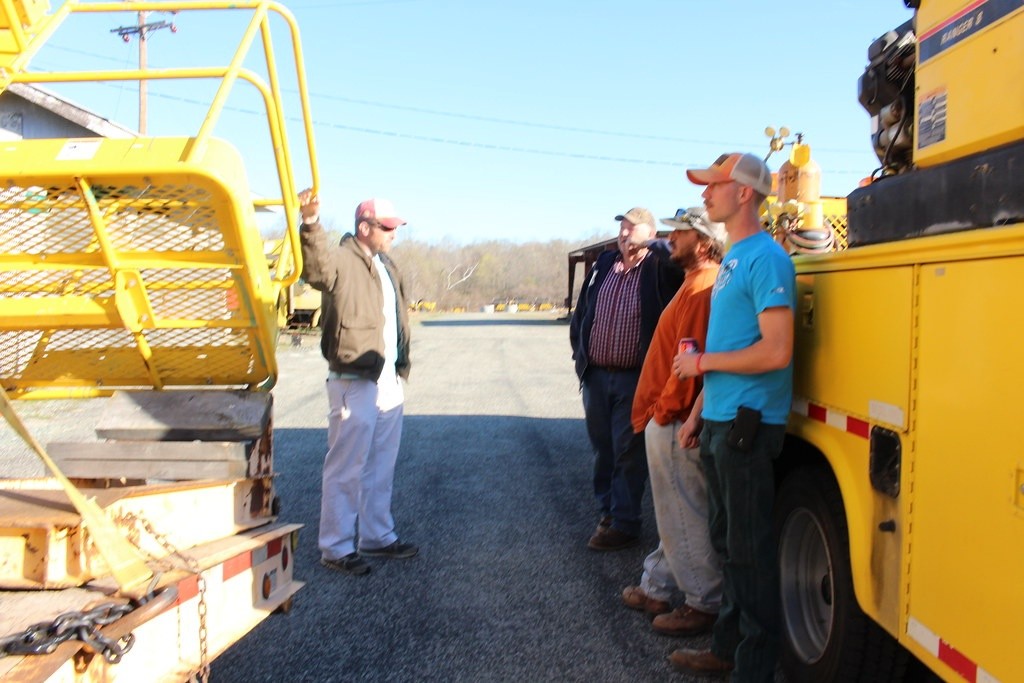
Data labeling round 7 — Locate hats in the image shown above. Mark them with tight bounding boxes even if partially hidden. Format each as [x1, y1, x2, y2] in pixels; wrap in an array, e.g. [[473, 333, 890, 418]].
[[661, 208, 726, 243], [686, 152, 773, 196], [615, 208, 656, 226], [358, 199, 407, 229]]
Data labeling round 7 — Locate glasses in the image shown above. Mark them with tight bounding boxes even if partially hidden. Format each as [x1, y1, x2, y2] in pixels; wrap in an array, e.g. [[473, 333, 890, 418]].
[[674, 208, 692, 225], [378, 225, 392, 231]]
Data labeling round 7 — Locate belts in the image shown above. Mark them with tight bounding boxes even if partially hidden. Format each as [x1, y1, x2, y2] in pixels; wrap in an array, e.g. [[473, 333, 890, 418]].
[[587, 365, 638, 373]]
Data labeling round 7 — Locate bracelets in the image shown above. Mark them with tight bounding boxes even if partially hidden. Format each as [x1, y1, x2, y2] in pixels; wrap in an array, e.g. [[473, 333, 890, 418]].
[[695, 352, 706, 374]]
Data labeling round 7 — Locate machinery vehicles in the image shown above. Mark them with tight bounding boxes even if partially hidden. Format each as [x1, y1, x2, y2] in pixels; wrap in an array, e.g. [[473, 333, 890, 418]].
[[0, 7, 330, 683], [566, 1, 1024, 683]]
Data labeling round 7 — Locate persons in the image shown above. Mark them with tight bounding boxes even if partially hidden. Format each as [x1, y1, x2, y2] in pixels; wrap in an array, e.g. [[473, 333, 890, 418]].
[[622, 206, 726, 637], [570, 208, 685, 551], [298, 186, 419, 576], [668, 152, 797, 682]]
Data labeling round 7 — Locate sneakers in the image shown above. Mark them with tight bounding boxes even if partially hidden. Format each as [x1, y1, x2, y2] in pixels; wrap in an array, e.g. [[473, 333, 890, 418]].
[[357, 536, 419, 557], [320, 551, 370, 574]]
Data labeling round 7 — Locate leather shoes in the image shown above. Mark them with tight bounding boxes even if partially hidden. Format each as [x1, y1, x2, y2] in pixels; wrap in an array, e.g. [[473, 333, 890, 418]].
[[587, 527, 639, 550], [652, 602, 717, 635], [594, 517, 612, 535], [671, 649, 734, 672], [623, 585, 668, 613]]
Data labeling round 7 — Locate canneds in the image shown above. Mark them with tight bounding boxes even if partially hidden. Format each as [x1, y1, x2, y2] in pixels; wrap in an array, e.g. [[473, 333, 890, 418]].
[[678, 338, 697, 355]]
